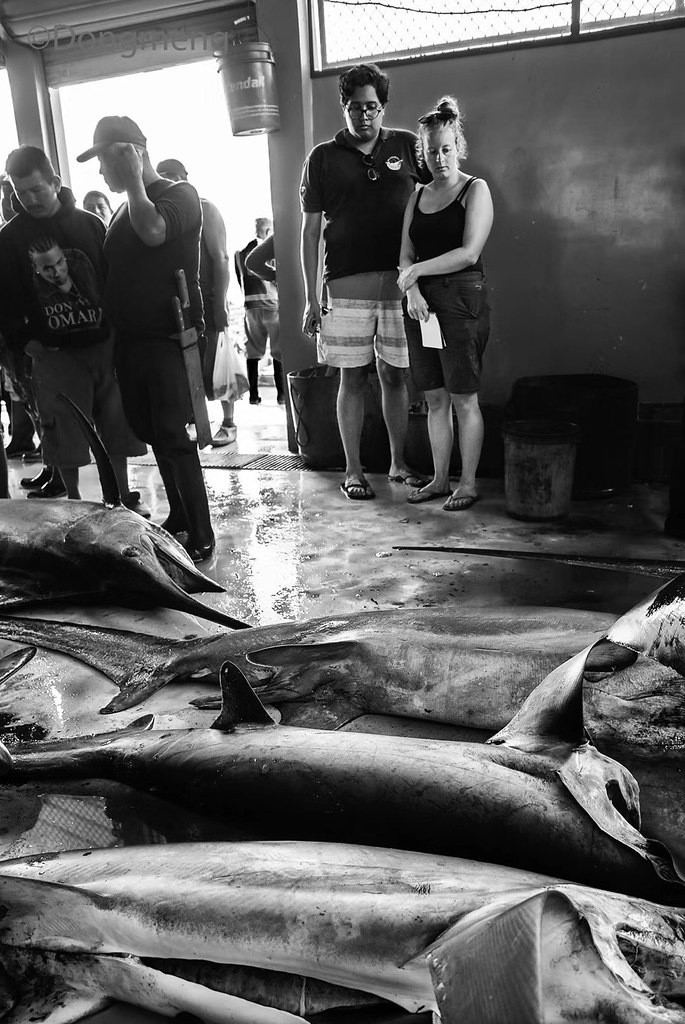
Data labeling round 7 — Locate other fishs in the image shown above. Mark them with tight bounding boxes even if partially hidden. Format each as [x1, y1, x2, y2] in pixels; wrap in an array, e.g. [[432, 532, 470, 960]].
[[0, 500, 255, 629], [0, 608, 685, 1024]]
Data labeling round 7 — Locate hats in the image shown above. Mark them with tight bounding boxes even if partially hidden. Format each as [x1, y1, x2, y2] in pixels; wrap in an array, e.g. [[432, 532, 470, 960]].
[[157, 159, 188, 180], [76, 116, 146, 162]]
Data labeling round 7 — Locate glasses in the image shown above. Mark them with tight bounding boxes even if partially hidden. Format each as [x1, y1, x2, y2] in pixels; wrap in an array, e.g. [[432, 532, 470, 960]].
[[417, 112, 456, 123], [363, 154, 380, 181], [343, 105, 386, 121]]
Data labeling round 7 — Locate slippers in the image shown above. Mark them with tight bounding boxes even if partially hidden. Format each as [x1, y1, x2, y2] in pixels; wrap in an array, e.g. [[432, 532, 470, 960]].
[[444, 490, 480, 511], [407, 486, 453, 504], [388, 470, 426, 488], [340, 475, 376, 500]]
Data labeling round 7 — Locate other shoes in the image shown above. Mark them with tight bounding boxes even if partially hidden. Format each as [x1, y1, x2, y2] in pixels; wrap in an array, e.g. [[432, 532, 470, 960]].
[[6, 443, 36, 457], [21, 468, 52, 489], [27, 479, 67, 498], [124, 492, 151, 519], [211, 426, 236, 445], [24, 443, 42, 462]]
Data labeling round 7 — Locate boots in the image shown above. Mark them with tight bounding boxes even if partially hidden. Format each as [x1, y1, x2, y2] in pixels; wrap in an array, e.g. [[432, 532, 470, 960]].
[[167, 441, 215, 560], [247, 359, 261, 404], [273, 357, 285, 404], [151, 447, 187, 537]]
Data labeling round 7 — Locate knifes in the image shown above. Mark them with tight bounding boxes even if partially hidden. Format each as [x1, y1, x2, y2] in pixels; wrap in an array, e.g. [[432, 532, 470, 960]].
[[172, 269, 195, 332]]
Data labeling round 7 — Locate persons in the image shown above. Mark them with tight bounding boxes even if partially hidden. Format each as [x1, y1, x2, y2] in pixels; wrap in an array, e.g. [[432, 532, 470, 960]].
[[0, 115, 277, 564], [300, 60, 494, 509]]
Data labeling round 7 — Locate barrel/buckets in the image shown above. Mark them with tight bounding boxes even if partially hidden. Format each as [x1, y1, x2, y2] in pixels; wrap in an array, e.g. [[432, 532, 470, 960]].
[[287, 366, 344, 465], [223, 42, 283, 135], [501, 421, 581, 519]]
